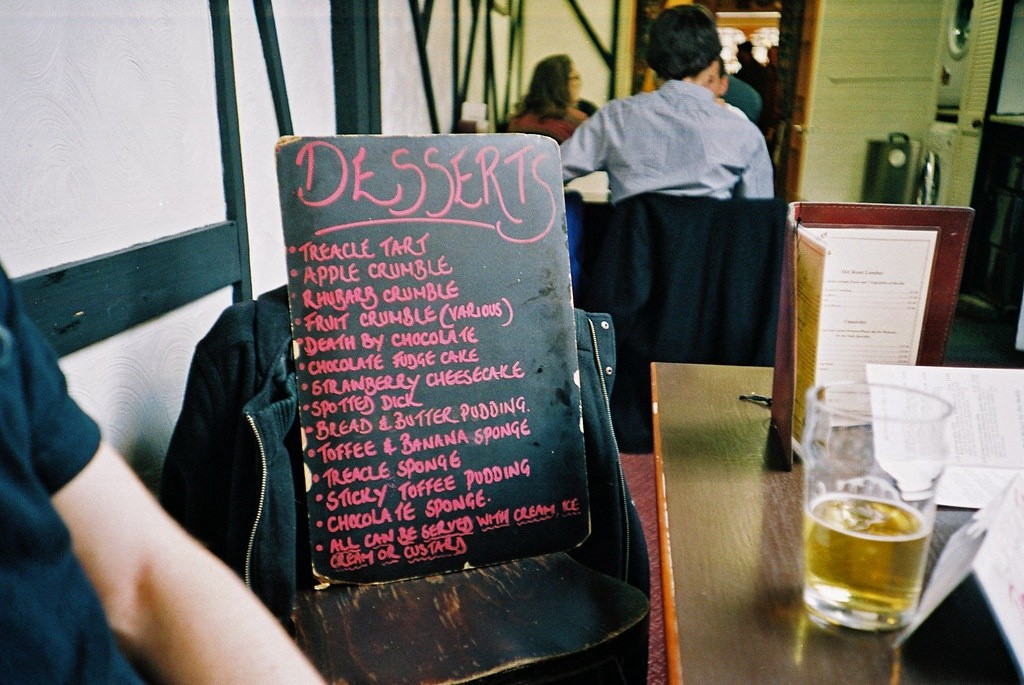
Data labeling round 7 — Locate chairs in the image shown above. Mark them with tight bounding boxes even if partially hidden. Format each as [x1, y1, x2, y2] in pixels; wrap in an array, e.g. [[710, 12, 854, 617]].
[[237, 285, 650, 685], [578, 193, 789, 455]]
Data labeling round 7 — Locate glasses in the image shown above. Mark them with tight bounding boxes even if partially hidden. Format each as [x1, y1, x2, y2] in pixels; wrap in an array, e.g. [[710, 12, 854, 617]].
[[567, 75, 579, 81]]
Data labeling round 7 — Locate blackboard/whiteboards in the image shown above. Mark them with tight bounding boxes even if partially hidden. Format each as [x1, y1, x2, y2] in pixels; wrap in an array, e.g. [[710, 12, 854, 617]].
[[278, 130, 594, 583]]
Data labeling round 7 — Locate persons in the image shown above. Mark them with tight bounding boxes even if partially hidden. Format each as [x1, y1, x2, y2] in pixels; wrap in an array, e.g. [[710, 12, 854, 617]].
[[0, 258, 327, 684], [559, 4, 775, 201], [505, 56, 588, 145], [704, 40, 778, 139]]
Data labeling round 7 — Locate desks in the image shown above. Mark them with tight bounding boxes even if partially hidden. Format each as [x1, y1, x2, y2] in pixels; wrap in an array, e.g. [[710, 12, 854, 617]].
[[651, 362, 982, 685]]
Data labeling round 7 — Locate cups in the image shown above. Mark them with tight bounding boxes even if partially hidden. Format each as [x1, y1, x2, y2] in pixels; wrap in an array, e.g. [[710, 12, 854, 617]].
[[802, 380, 954, 632]]
